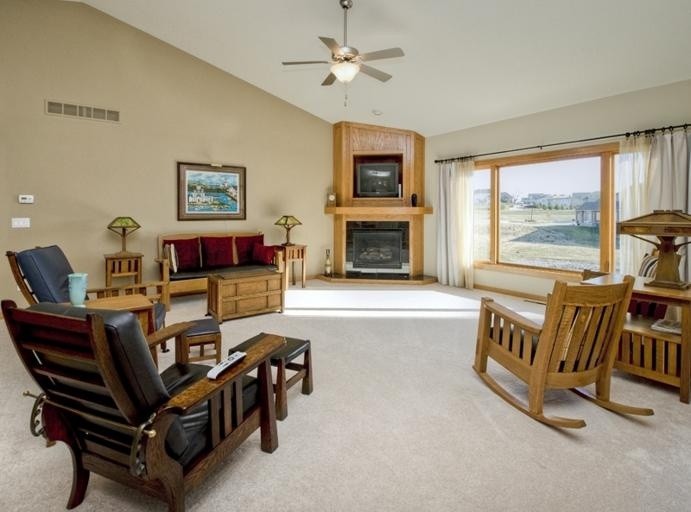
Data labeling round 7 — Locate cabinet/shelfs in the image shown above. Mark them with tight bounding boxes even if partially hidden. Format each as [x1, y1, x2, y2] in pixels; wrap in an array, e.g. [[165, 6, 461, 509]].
[[205, 268, 286, 324]]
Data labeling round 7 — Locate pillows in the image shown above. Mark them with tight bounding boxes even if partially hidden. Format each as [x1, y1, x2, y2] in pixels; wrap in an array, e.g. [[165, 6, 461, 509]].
[[638, 253, 659, 277], [162, 234, 276, 273]]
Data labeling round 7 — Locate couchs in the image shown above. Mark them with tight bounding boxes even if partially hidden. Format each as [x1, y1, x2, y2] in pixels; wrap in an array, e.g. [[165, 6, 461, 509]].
[[154, 230, 290, 312]]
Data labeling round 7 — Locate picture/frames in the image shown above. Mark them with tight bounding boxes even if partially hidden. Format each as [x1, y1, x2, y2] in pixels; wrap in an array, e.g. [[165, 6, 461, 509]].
[[177, 161, 246, 222]]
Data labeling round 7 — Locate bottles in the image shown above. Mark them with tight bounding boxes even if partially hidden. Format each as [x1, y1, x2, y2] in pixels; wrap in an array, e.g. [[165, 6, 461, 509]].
[[412, 193, 417, 207], [324, 248, 333, 277]]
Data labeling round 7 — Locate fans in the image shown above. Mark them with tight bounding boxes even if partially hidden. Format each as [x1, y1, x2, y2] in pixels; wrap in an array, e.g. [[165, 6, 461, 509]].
[[282, 1, 405, 86]]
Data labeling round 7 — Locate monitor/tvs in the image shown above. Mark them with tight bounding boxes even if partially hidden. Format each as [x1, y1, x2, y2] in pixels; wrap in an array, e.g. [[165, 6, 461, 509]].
[[356, 163, 399, 196]]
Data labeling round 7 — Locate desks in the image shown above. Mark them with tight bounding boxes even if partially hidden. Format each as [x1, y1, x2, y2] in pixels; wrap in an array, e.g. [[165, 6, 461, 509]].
[[104, 252, 143, 287], [276, 244, 308, 290], [580, 274, 691, 404]]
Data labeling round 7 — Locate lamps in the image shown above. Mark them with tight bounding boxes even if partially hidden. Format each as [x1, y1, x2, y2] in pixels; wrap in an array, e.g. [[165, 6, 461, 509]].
[[107, 217, 140, 253], [330, 60, 361, 83], [616, 209, 691, 291], [274, 215, 302, 246]]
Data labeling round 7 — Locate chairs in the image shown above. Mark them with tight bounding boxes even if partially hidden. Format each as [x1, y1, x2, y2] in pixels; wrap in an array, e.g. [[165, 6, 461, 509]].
[[583, 249, 682, 320], [472, 275, 654, 429], [1, 299, 279, 512], [6, 245, 170, 353]]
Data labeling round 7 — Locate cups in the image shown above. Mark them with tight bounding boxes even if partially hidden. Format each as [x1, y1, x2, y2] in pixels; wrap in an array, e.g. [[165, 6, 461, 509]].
[[66, 272, 89, 308]]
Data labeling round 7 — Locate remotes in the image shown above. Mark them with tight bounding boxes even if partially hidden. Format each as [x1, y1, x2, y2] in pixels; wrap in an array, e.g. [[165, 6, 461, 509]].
[[207, 351, 247, 380]]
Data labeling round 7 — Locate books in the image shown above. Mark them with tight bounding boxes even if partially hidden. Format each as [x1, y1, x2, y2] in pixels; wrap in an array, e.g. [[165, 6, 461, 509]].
[[651, 318, 682, 335]]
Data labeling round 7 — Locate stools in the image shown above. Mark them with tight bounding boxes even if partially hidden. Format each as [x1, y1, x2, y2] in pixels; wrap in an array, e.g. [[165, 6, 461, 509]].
[[228, 332, 313, 421], [186, 318, 221, 365]]
